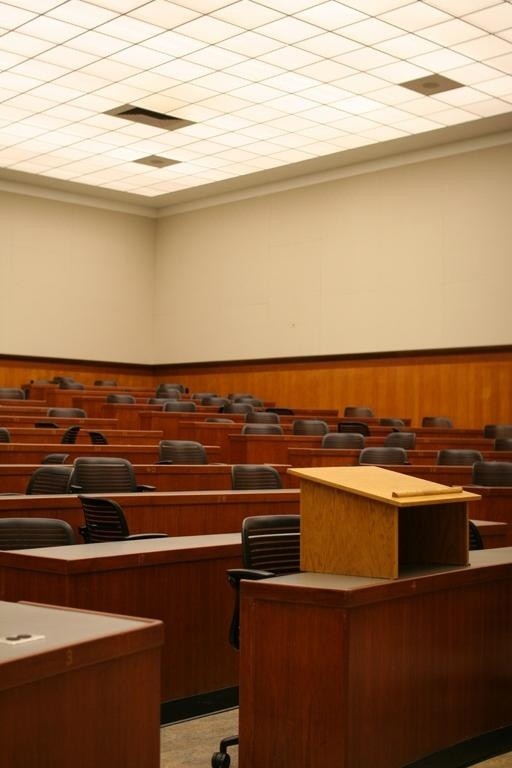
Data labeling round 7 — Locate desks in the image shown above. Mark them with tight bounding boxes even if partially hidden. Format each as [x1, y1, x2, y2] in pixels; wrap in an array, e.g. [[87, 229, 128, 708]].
[[288, 448, 512, 488], [0, 600, 165, 767], [360, 463, 472, 486], [1, 464, 291, 495], [0, 399, 47, 407], [100, 403, 338, 429], [0, 488, 300, 543], [140, 411, 412, 440], [49, 388, 194, 408], [181, 421, 485, 462], [455, 484, 510, 548], [229, 434, 511, 465], [0, 442, 221, 464], [73, 395, 276, 419], [1, 415, 119, 430], [240, 546, 511, 767], [0, 405, 56, 416], [1, 428, 162, 444], [29, 384, 189, 401], [0, 518, 510, 725]]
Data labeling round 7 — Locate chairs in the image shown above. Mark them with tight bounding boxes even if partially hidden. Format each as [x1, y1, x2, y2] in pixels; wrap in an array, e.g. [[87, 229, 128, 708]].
[[94, 381, 117, 386], [345, 408, 372, 418], [469, 520, 485, 550], [59, 383, 84, 390], [60, 425, 81, 444], [219, 402, 254, 414], [435, 450, 483, 466], [71, 457, 157, 493], [0, 388, 25, 400], [48, 408, 85, 417], [201, 397, 229, 406], [244, 412, 280, 423], [205, 418, 234, 423], [147, 397, 179, 404], [484, 424, 512, 438], [228, 396, 253, 402], [231, 464, 283, 490], [0, 427, 12, 443], [265, 409, 294, 415], [79, 496, 169, 543], [1, 518, 75, 548], [358, 447, 409, 465], [0, 466, 75, 496], [291, 419, 329, 436], [338, 422, 370, 437], [107, 395, 136, 404], [384, 433, 415, 449], [242, 424, 283, 434], [212, 515, 300, 758], [422, 417, 451, 427], [162, 402, 197, 412], [321, 433, 365, 448], [494, 439, 512, 450], [192, 394, 220, 402], [378, 418, 404, 425], [235, 399, 262, 407], [157, 389, 181, 398], [34, 422, 62, 427], [88, 431, 108, 444], [155, 440, 208, 465], [41, 454, 70, 464], [49, 377, 75, 384], [471, 462, 511, 487], [392, 427, 404, 432], [156, 383, 186, 392]]
